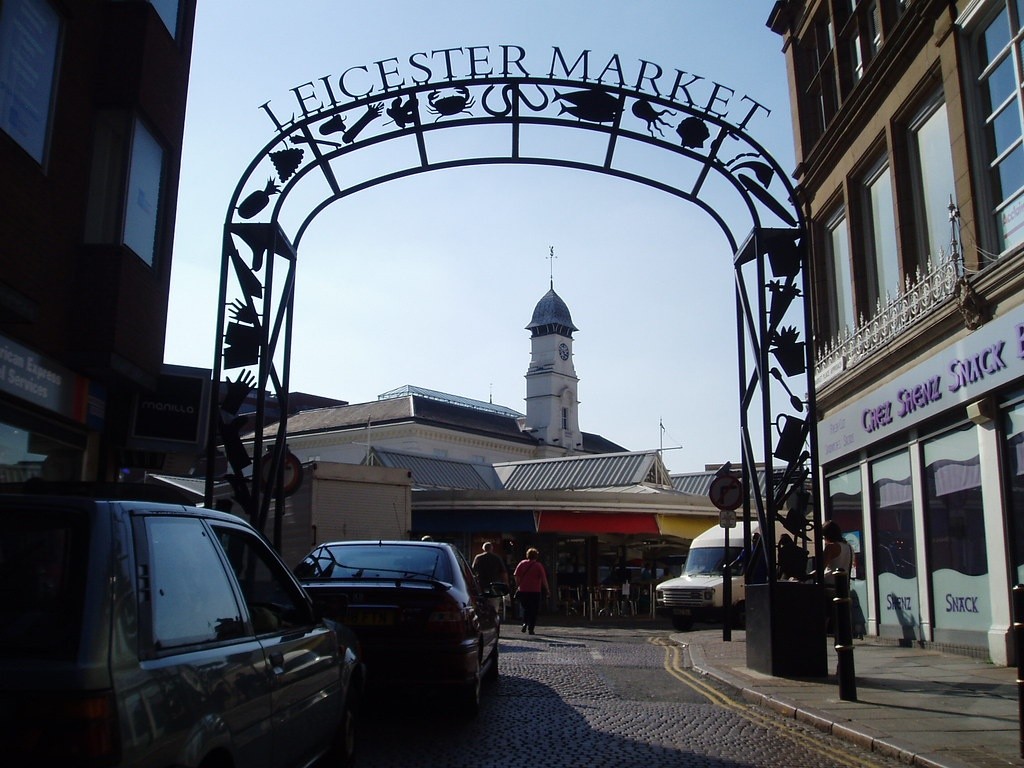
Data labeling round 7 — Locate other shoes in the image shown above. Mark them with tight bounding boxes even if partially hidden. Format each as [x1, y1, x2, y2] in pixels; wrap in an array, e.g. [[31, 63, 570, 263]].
[[521, 624, 527, 632], [529, 630, 535, 635]]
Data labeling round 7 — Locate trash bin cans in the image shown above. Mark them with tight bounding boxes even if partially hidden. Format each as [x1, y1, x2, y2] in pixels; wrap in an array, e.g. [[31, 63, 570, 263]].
[[743, 581, 829, 679]]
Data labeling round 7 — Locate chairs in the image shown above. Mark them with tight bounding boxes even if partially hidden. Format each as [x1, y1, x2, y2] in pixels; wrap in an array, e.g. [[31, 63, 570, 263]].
[[557, 584, 643, 620]]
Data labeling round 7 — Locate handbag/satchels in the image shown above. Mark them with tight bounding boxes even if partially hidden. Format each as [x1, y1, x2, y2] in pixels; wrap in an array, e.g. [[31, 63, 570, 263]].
[[514, 589, 520, 600]]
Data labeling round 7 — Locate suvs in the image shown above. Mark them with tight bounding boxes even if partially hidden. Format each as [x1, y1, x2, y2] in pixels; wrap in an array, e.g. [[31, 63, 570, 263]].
[[0, 494, 374, 768], [292, 536, 509, 733]]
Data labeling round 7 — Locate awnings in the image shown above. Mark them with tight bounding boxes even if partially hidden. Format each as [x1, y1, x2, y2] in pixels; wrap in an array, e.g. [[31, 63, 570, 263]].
[[410, 509, 538, 534], [538, 511, 659, 537], [654, 515, 721, 540]]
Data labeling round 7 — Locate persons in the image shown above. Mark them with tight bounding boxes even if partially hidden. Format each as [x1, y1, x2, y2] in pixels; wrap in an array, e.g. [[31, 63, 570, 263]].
[[513, 548, 549, 635], [751, 533, 769, 584], [777, 534, 804, 580], [472, 541, 511, 613], [821, 521, 852, 590], [849, 590, 866, 639]]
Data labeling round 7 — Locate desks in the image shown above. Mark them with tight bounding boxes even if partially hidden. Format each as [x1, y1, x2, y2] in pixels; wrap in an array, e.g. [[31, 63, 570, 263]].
[[595, 587, 621, 618]]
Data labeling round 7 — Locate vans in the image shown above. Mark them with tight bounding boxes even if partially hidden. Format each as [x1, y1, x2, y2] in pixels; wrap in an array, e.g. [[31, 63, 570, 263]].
[[656, 520, 817, 631]]
